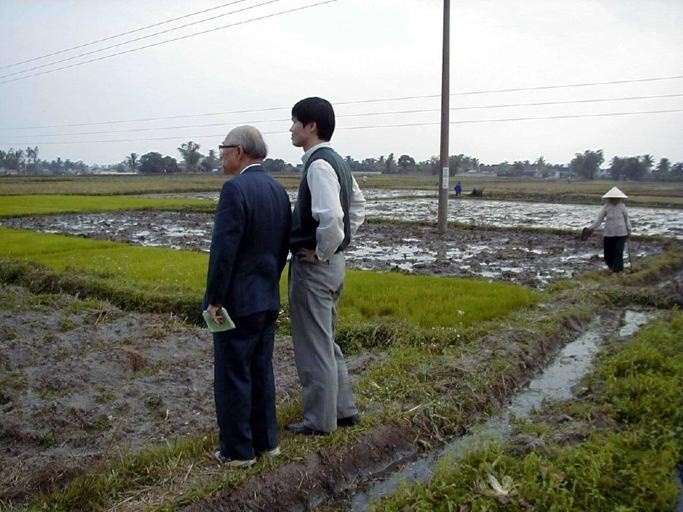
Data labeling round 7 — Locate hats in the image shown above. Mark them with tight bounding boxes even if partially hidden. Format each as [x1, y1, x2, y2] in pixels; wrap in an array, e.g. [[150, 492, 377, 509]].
[[602, 186, 629, 198]]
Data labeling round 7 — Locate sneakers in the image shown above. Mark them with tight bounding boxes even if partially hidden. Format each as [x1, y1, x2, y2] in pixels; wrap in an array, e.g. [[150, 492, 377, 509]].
[[215, 445, 281, 467]]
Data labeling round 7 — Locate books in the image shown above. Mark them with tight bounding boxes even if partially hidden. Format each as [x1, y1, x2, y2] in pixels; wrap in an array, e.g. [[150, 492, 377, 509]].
[[201, 306, 237, 333]]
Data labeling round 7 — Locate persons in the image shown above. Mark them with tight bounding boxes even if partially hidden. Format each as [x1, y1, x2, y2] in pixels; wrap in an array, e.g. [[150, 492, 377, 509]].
[[453, 180, 463, 195], [200, 122, 293, 470], [286, 94, 366, 436], [584, 185, 631, 271]]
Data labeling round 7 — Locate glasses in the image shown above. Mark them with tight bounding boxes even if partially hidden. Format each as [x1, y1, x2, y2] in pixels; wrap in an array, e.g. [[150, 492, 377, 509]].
[[219, 145, 239, 152]]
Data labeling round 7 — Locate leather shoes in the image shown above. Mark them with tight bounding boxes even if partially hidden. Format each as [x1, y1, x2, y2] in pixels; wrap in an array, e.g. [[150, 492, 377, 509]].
[[286, 413, 359, 436]]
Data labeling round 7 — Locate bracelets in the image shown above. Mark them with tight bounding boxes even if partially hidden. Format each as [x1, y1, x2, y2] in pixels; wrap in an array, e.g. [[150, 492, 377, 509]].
[[314, 250, 318, 260]]
